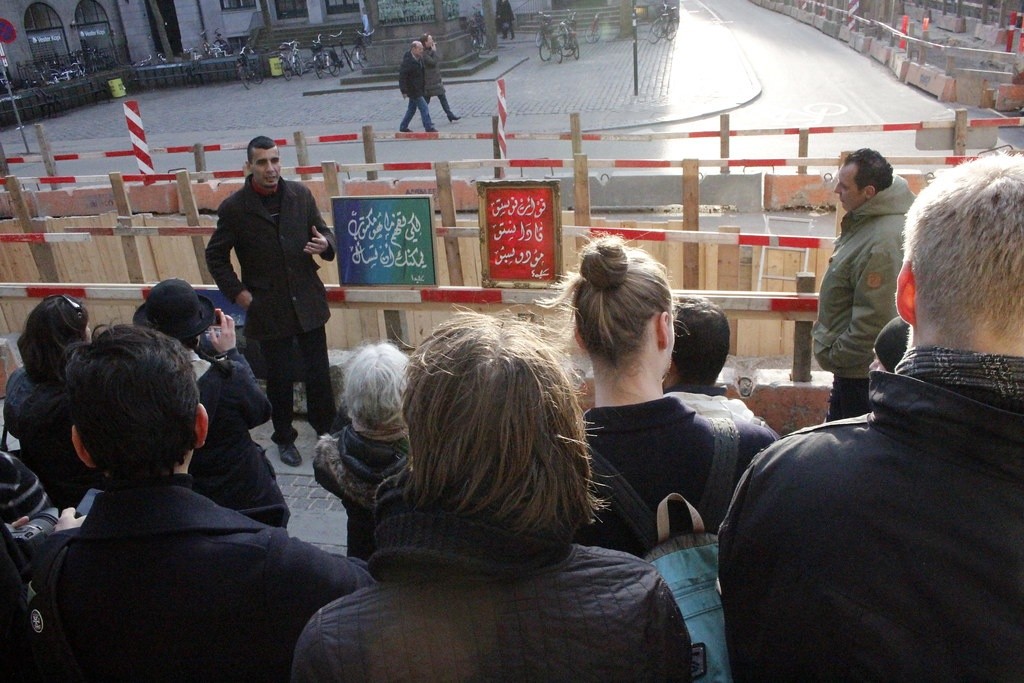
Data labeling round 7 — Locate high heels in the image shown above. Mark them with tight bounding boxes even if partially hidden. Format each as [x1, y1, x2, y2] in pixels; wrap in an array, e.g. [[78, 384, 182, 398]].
[[448, 115, 460, 122]]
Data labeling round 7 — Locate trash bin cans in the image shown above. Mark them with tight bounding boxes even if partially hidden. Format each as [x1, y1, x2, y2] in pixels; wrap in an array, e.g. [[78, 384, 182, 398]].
[[106, 76, 127, 98], [268, 55, 283, 77]]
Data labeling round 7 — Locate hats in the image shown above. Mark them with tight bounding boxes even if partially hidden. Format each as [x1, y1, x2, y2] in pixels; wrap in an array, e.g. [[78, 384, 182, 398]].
[[132, 280, 214, 340], [874, 316, 910, 373]]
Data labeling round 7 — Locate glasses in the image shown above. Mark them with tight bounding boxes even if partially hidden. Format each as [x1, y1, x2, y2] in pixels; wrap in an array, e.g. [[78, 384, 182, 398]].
[[44, 293, 85, 338]]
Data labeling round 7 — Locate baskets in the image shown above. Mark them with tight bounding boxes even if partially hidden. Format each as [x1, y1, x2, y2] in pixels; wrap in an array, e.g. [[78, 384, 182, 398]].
[[310, 44, 322, 53]]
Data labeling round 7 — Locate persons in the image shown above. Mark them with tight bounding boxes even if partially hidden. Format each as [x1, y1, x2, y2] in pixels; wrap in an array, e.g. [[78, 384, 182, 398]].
[[810, 148, 917, 424], [718, 152, 1023, 683], [497, 0, 516, 40], [544, 234, 781, 683], [399, 41, 439, 132], [205, 136, 337, 467], [419, 33, 461, 125], [662, 294, 781, 525], [312, 342, 410, 562], [0, 278, 291, 683], [15, 325, 377, 683], [868, 317, 910, 373], [292, 309, 691, 683]]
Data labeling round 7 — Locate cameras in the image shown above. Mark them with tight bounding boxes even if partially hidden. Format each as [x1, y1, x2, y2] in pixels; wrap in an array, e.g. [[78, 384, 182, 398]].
[[4, 506, 61, 548]]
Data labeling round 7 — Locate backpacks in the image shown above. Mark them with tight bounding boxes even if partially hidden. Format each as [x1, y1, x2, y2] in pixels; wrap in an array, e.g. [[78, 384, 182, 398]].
[[575, 417, 736, 683]]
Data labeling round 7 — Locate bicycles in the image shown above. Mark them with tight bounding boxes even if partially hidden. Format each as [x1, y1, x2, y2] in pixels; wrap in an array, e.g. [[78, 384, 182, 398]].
[[469, 23, 483, 51], [180, 28, 234, 76], [272, 39, 303, 82], [17, 49, 116, 89], [538, 9, 579, 64], [584, 12, 602, 44], [310, 29, 373, 79], [235, 39, 263, 90], [646, 2, 679, 44]]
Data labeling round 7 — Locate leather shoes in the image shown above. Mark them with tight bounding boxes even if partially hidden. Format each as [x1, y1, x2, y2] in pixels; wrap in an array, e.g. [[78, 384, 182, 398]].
[[277, 443, 303, 468]]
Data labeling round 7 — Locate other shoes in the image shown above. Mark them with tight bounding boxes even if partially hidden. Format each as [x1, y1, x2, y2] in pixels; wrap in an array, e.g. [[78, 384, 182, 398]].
[[427, 128, 439, 134], [400, 128, 412, 132]]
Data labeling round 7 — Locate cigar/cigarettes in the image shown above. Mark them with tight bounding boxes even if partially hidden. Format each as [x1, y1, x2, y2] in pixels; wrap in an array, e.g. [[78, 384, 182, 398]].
[[434, 43, 437, 44]]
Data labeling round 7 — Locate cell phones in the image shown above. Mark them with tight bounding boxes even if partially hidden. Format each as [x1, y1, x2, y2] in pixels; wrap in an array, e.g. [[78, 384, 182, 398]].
[[205, 312, 222, 341], [75, 489, 104, 519]]
